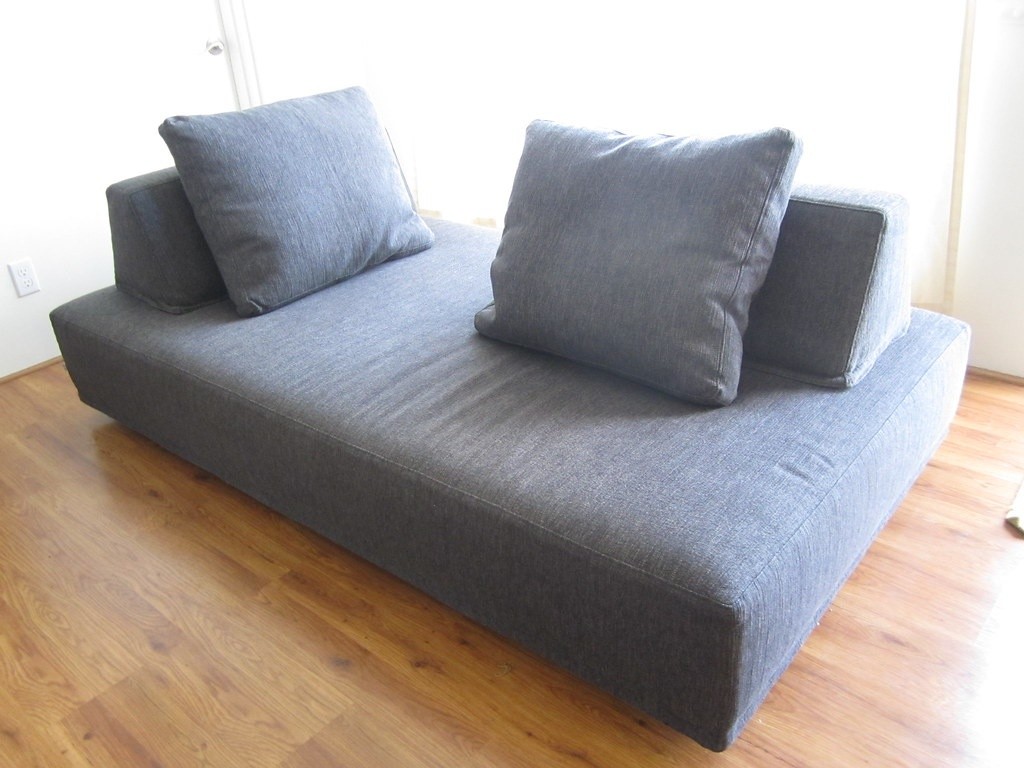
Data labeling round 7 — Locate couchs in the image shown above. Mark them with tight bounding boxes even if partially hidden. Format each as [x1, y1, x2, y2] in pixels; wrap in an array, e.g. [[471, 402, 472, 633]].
[[47, 167, 972, 754]]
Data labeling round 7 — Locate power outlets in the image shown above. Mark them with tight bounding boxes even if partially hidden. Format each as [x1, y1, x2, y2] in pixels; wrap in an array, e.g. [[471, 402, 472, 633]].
[[6, 258, 42, 297]]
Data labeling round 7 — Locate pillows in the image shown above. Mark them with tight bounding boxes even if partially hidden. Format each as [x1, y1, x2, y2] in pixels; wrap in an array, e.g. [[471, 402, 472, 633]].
[[158, 86, 435, 320], [473, 120, 803, 410]]
